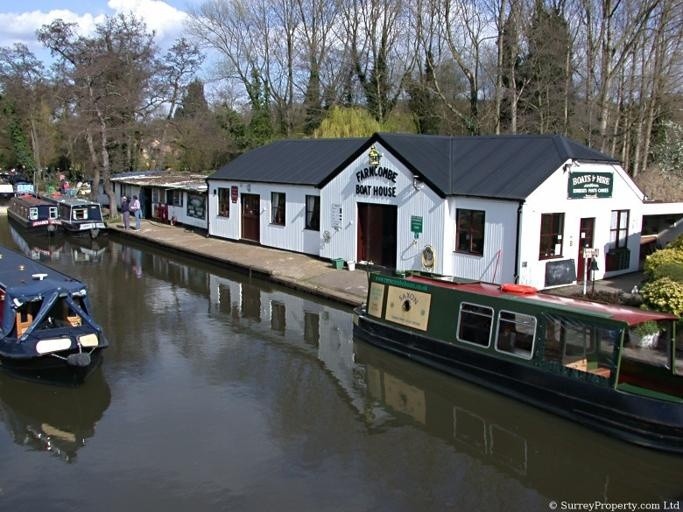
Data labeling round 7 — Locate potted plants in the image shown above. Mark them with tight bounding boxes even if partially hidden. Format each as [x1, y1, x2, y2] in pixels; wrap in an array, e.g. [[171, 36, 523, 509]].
[[629, 322, 660, 348]]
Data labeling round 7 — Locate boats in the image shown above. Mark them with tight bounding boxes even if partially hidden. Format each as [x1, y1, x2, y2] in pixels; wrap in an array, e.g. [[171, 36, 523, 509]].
[[0, 172, 92, 198], [356, 344, 683, 512], [0, 369, 115, 464], [8, 220, 64, 263], [41, 192, 107, 238], [65, 233, 108, 266], [0, 245, 111, 391], [5, 194, 62, 234], [348, 260, 683, 456]]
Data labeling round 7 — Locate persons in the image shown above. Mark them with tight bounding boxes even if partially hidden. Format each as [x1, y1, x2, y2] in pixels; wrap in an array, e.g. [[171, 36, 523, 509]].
[[121, 195, 142, 231]]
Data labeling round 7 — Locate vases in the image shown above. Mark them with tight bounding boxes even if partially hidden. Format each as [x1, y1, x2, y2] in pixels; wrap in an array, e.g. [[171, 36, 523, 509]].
[[333, 258, 344, 269], [347, 260, 356, 271]]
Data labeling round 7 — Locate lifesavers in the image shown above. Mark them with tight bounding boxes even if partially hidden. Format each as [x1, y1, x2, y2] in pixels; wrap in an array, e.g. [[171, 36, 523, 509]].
[[499, 284, 538, 295]]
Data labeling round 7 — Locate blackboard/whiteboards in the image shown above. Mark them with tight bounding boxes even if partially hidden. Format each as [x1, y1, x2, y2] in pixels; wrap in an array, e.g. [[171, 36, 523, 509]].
[[545, 259, 577, 287]]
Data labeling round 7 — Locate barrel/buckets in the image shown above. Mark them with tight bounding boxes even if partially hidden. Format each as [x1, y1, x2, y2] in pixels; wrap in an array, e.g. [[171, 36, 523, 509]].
[[347, 259, 355, 271], [334, 258, 344, 270]]
